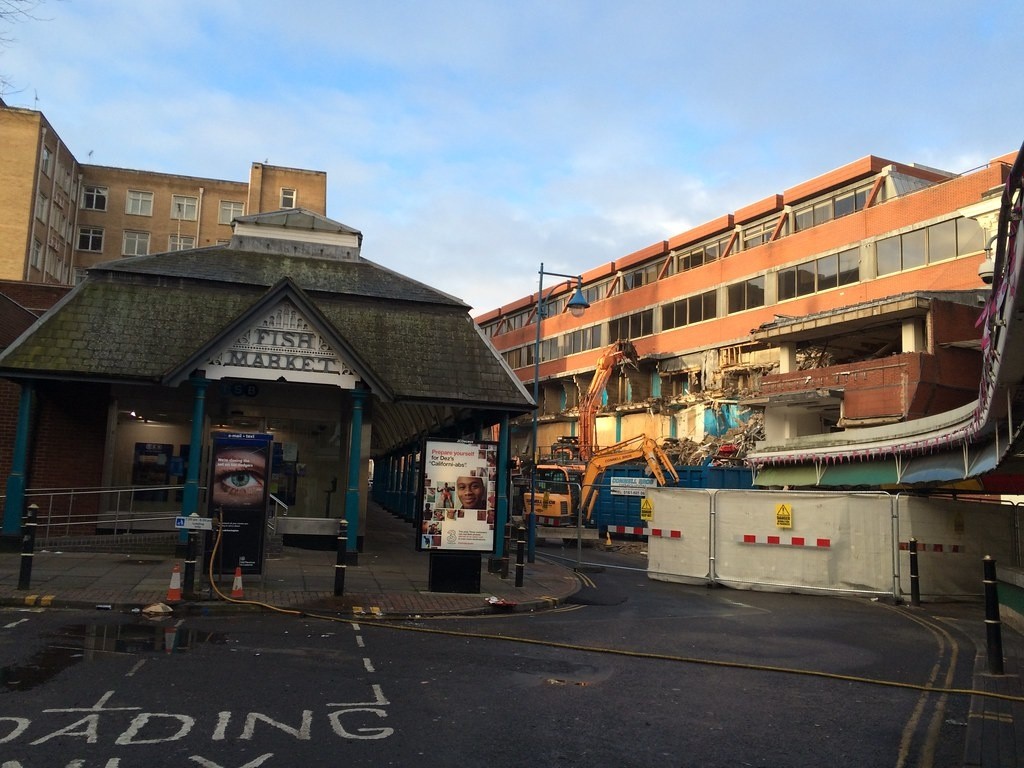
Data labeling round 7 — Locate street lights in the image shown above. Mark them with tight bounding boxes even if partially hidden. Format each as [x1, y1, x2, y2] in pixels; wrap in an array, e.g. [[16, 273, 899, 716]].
[[526, 262, 593, 564]]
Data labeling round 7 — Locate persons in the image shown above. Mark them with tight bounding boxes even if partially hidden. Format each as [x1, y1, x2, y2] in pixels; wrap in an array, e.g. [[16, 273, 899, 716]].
[[477, 467, 488, 477], [488, 492, 495, 509], [456, 477, 487, 510], [422, 502, 434, 521], [486, 451, 496, 467], [423, 536, 431, 549], [435, 481, 455, 509], [488, 468, 497, 481], [423, 522, 442, 535], [488, 510, 494, 524], [434, 509, 445, 521], [211, 444, 266, 506]]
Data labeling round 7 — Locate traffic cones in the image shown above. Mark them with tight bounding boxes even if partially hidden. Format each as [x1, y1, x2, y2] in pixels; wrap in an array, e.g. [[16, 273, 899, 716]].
[[227, 567, 248, 605], [165, 564, 188, 606]]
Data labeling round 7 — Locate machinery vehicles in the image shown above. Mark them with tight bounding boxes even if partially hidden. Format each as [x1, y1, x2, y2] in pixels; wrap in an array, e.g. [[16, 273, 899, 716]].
[[512, 340, 681, 529]]
